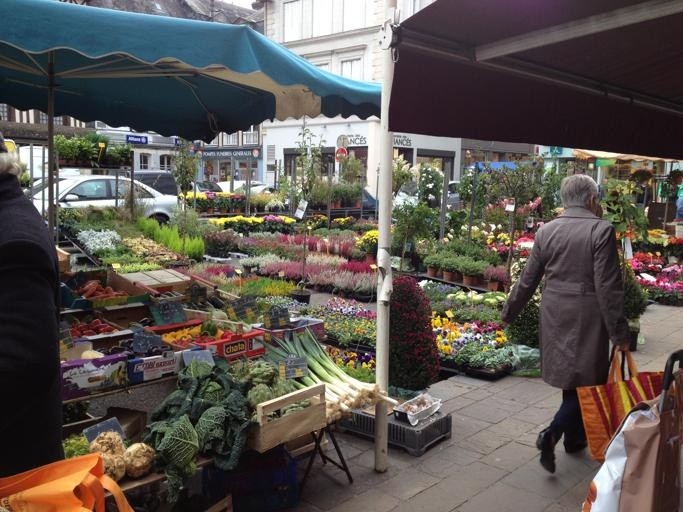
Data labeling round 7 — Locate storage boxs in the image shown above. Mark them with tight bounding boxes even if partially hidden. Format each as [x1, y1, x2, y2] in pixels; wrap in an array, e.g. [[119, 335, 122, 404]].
[[48, 266, 327, 401]]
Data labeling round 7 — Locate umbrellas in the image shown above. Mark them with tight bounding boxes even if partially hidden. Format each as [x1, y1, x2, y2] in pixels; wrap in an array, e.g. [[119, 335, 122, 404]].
[[0, 0, 382, 244]]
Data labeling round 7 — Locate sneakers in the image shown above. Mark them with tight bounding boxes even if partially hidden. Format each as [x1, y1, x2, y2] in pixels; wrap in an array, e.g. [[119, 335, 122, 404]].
[[536, 425, 557, 474], [563, 440, 587, 454]]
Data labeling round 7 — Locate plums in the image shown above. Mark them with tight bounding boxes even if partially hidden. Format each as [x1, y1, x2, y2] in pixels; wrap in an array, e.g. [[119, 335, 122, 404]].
[[95, 337, 171, 359]]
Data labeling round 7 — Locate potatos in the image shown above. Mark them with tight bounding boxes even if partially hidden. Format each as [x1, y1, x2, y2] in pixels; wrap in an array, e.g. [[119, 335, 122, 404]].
[[75, 279, 130, 301]]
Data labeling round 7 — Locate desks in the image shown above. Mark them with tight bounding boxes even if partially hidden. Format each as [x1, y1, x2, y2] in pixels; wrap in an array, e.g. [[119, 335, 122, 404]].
[[96, 410, 359, 512]]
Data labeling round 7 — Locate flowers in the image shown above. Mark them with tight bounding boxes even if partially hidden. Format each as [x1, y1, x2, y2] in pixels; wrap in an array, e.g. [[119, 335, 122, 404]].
[[626, 228, 683, 305], [206, 214, 357, 234], [56, 206, 177, 271], [355, 229, 378, 252], [447, 220, 511, 254], [307, 278, 513, 388], [176, 191, 246, 208]]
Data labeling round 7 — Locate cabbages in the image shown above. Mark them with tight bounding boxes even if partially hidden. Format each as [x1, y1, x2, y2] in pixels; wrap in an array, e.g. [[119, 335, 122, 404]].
[[145, 357, 250, 485]]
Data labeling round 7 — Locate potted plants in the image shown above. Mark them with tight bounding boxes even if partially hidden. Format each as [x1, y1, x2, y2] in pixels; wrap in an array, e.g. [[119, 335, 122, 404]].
[[326, 174, 361, 208], [49, 135, 99, 167], [423, 248, 507, 289]]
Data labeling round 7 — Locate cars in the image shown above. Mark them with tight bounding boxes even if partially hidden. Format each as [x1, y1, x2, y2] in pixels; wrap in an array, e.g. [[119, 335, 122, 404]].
[[180, 180, 224, 208], [235, 184, 279, 194], [361, 187, 379, 208], [23, 167, 178, 225], [393, 181, 461, 209]]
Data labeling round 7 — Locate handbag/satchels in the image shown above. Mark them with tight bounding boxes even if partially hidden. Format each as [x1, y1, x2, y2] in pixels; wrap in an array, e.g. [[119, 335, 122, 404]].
[[576, 345, 665, 464]]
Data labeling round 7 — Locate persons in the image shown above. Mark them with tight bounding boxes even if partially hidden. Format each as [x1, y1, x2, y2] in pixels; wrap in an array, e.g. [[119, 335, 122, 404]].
[[0, 131, 65, 478], [500, 174, 630, 473], [636, 180, 652, 208]]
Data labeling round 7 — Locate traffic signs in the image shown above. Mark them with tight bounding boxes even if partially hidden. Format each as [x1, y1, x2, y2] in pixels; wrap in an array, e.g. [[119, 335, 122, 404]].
[[335, 147, 348, 162]]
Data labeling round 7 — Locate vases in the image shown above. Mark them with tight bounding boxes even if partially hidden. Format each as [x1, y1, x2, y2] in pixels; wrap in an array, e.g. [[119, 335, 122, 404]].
[[196, 206, 240, 215], [367, 254, 375, 264]]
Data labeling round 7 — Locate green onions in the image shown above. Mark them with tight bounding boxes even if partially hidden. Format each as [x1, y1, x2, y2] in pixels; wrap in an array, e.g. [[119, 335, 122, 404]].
[[254, 324, 399, 425]]
